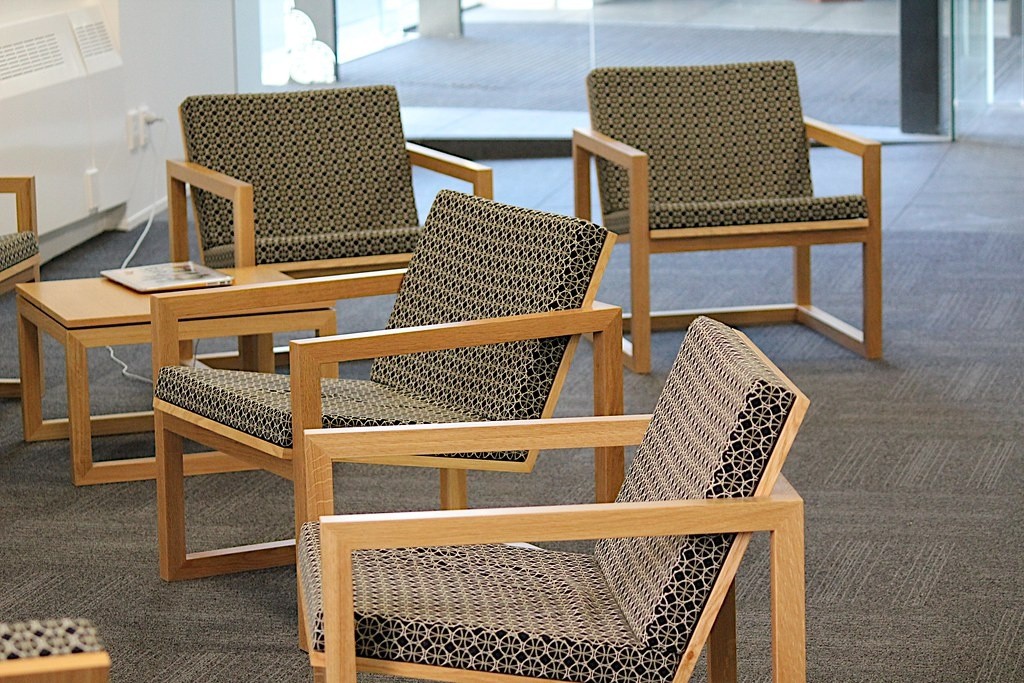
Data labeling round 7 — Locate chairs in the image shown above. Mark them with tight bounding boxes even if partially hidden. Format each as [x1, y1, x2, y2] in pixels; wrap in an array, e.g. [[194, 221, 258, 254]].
[[167, 81, 501, 372], [571, 59, 893, 373], [0, 175, 45, 397], [306, 317, 811, 681]]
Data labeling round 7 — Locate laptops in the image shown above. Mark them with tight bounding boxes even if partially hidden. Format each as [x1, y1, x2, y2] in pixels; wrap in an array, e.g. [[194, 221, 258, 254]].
[[99, 261, 233, 292]]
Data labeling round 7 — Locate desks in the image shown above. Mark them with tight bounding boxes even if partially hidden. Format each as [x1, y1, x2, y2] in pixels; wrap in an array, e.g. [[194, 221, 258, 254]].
[[145, 187, 616, 582], [13, 264, 340, 486]]
